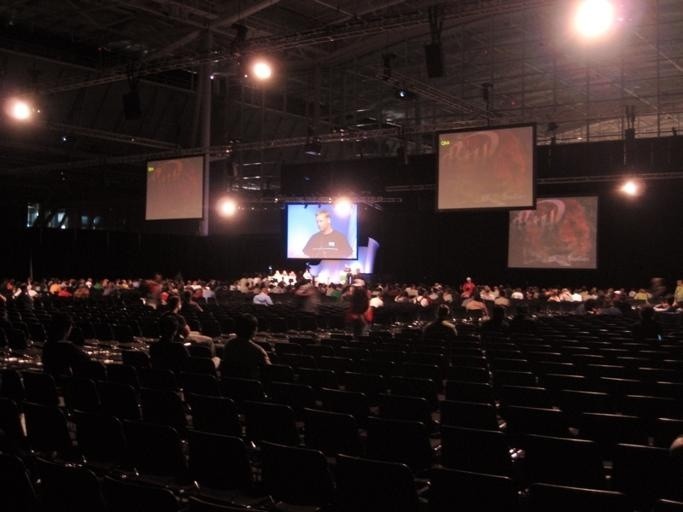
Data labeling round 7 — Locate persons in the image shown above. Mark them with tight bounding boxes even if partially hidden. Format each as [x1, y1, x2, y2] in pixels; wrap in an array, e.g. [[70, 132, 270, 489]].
[[299, 210, 353, 258], [1, 264, 682, 392]]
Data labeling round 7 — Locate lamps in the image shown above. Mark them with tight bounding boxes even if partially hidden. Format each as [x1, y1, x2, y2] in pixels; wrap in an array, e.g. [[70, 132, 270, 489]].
[[426, 6, 444, 77], [305, 137, 322, 156], [623, 106, 636, 165], [123, 62, 145, 120], [230, 22, 248, 56], [396, 90, 417, 101]]
